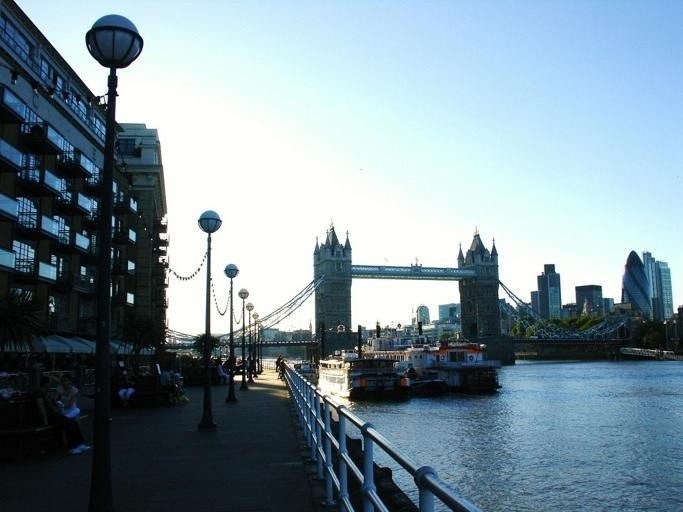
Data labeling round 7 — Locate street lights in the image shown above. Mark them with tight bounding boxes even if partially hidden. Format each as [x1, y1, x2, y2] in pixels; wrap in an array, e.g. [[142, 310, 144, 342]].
[[87, 14, 143, 512], [224, 264, 238, 401], [245, 303, 264, 383], [198, 209, 222, 429], [240, 288, 249, 391]]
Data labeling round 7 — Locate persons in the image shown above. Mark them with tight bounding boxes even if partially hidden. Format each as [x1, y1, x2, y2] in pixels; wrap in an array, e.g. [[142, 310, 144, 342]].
[[52, 374, 91, 420], [275, 354, 287, 379], [37, 377, 91, 455], [210, 356, 250, 385]]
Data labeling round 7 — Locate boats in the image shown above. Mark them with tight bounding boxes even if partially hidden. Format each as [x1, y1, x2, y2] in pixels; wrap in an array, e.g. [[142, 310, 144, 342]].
[[348, 358, 411, 400], [320, 360, 346, 378], [294, 356, 319, 386], [402, 338, 502, 393]]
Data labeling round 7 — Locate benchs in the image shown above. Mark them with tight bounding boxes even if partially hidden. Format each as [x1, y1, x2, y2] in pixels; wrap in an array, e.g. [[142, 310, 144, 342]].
[[111, 384, 173, 408], [0, 401, 87, 464]]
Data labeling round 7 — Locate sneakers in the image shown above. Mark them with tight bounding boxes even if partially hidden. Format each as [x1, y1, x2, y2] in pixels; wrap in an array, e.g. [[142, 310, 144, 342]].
[[78, 444, 91, 452], [70, 448, 82, 455]]
